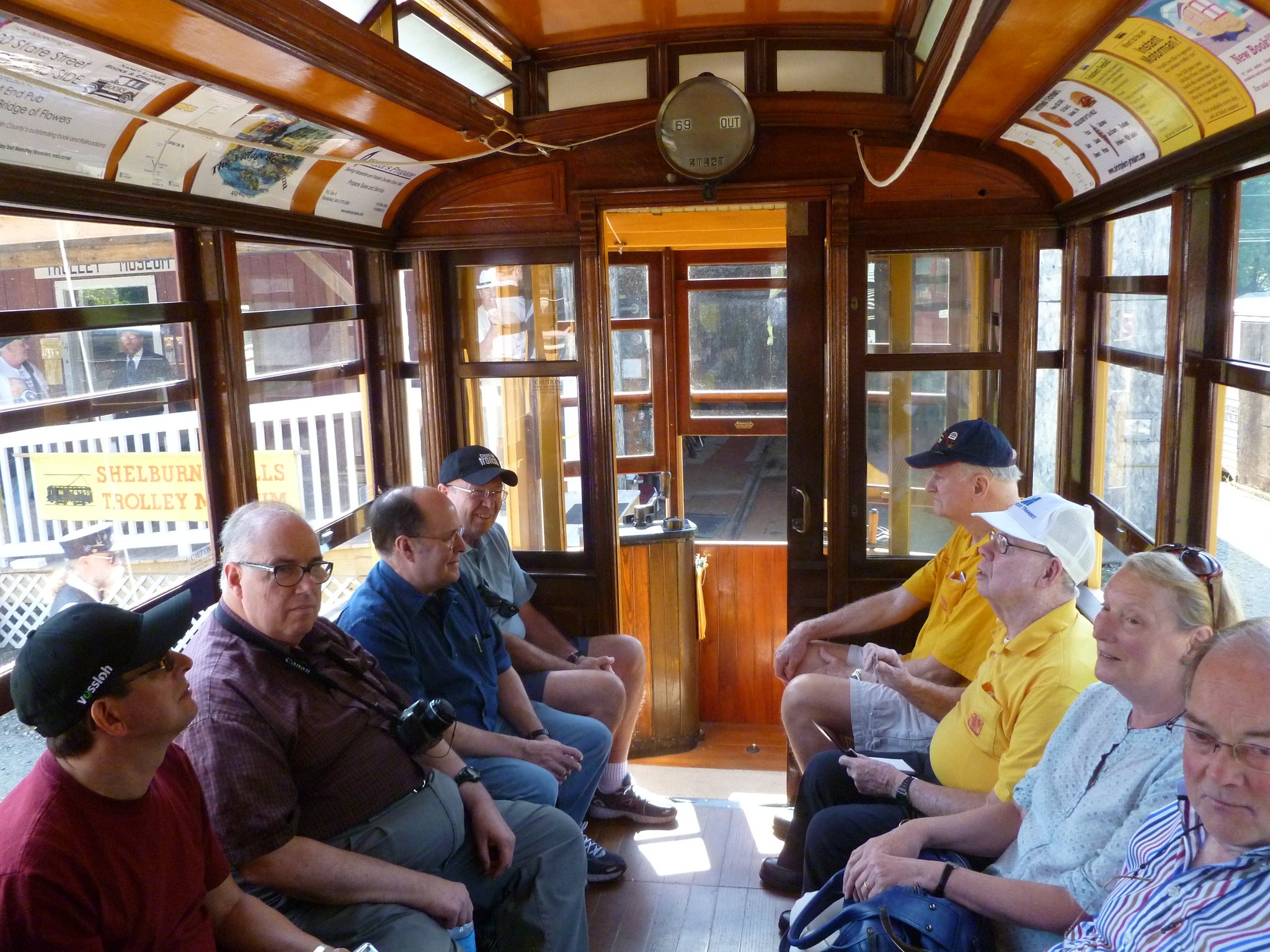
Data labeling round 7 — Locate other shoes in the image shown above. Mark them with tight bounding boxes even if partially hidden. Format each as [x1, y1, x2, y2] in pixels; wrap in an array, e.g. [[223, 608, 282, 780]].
[[778, 909, 791, 931]]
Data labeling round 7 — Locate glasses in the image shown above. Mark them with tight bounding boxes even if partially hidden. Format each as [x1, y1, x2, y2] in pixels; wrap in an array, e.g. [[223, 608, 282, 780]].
[[236, 561, 334, 587], [90, 555, 116, 565], [407, 527, 465, 550], [446, 485, 508, 502], [988, 529, 1054, 556], [1166, 709, 1270, 774], [90, 648, 174, 732], [1147, 543, 1221, 627], [120, 334, 138, 341]]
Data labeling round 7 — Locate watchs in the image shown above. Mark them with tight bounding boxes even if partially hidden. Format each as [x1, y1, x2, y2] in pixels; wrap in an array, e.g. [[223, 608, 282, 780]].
[[454, 765, 482, 788], [935, 861, 958, 897], [896, 776, 918, 806], [852, 667, 863, 681]]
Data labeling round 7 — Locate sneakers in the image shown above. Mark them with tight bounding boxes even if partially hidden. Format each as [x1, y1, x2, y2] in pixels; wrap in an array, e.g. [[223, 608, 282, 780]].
[[758, 857, 804, 893], [587, 773, 677, 824], [773, 811, 793, 838], [581, 821, 627, 881]]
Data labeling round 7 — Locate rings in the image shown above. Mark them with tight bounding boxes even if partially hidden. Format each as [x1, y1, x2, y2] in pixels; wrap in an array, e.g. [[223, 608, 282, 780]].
[[864, 881, 871, 891]]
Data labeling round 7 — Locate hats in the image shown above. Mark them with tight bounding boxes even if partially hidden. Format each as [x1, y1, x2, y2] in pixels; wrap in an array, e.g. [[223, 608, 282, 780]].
[[10, 589, 193, 738], [971, 492, 1096, 586], [905, 418, 1017, 469], [128, 358, 136, 378], [0, 336, 22, 348], [59, 522, 113, 560], [439, 445, 518, 487]]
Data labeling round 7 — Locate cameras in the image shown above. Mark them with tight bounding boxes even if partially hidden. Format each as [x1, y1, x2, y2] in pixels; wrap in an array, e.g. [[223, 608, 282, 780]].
[[390, 698, 457, 754]]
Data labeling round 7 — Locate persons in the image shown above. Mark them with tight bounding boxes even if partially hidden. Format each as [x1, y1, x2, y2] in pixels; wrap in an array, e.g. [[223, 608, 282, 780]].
[[0, 335, 55, 542], [1045, 615, 1270, 952], [774, 418, 1024, 831], [109, 329, 176, 451], [173, 500, 588, 952], [0, 589, 349, 952], [842, 542, 1245, 952], [337, 486, 626, 881], [438, 444, 677, 823], [759, 492, 1098, 933], [49, 521, 122, 617], [475, 267, 505, 452]]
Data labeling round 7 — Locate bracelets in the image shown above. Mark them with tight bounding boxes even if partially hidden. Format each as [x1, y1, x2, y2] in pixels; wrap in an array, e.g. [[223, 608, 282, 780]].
[[313, 943, 329, 952], [526, 728, 551, 740], [567, 651, 583, 663]]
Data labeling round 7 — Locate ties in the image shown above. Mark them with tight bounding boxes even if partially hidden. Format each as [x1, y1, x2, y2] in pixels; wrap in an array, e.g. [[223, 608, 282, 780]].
[[100, 590, 104, 602]]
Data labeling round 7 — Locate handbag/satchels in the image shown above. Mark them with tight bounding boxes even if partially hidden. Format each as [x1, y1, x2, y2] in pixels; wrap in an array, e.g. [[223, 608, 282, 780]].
[[779, 814, 1003, 952]]
